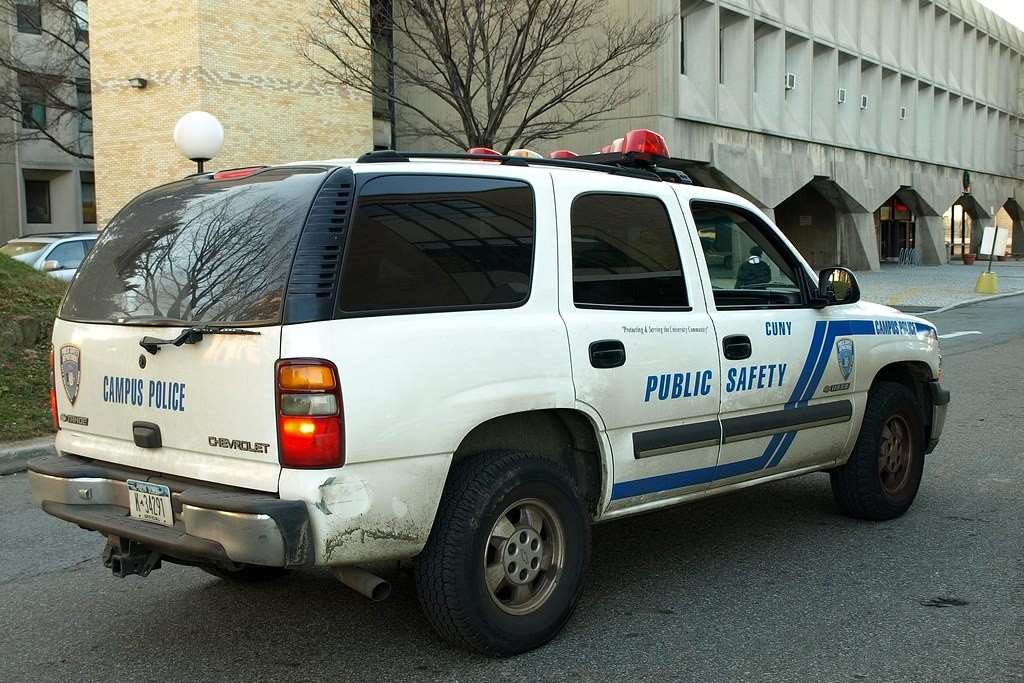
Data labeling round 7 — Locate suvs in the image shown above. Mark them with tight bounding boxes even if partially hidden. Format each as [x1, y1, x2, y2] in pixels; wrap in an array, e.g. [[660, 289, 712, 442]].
[[26, 129, 954, 659]]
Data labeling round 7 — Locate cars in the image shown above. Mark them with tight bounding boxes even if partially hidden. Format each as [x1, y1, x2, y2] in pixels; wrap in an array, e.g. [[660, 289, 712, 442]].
[[0, 230, 105, 283]]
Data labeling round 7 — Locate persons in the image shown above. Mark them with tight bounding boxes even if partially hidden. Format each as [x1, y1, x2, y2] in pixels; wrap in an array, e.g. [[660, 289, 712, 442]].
[[734, 246, 771, 290]]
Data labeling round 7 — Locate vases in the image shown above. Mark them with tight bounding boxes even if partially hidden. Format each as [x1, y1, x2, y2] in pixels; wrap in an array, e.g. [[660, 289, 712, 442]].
[[962, 253, 976, 264]]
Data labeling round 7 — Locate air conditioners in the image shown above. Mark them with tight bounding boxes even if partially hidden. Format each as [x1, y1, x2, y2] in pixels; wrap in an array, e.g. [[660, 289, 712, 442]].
[[900, 106, 905, 120], [861, 95, 867, 110], [837, 89, 845, 104], [785, 73, 795, 90]]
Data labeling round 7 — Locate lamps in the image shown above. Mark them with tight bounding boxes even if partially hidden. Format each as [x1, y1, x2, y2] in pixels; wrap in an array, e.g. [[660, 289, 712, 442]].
[[128, 78, 147, 89]]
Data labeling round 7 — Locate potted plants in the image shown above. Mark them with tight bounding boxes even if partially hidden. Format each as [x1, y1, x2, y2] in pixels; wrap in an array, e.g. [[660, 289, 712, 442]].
[[997, 252, 1008, 261]]
